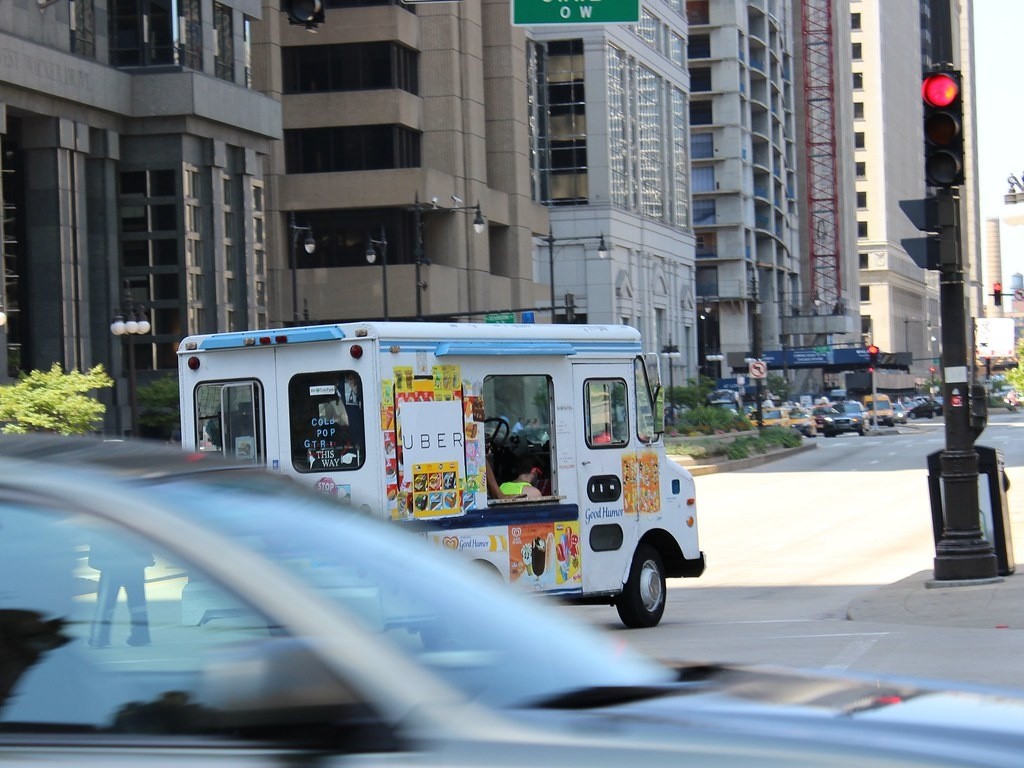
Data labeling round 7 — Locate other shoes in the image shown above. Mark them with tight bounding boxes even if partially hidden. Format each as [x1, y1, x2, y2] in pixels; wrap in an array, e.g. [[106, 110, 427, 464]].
[[125, 638, 150, 648], [85, 640, 109, 649]]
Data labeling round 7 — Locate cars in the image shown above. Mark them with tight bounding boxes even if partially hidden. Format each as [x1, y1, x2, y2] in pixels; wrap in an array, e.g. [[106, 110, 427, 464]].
[[703, 384, 945, 437], [822, 400, 871, 437], [0, 429, 1022, 768]]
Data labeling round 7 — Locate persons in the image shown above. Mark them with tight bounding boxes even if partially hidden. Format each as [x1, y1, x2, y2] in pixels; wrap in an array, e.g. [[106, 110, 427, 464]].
[[496, 412, 539, 433], [485, 459, 520, 498], [499, 458, 542, 497], [86, 529, 156, 647]]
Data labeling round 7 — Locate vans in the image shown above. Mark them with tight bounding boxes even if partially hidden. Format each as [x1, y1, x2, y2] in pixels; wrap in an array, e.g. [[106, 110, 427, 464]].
[[173, 318, 707, 629]]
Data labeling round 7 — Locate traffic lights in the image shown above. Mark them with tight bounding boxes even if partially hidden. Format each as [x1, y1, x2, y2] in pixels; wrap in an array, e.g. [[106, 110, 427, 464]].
[[994, 282, 1002, 306], [919, 70, 966, 190], [897, 194, 957, 272], [868, 346, 877, 366]]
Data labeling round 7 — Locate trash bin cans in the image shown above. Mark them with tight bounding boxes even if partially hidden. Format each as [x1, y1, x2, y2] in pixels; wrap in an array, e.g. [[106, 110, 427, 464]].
[[927, 446, 1015, 577]]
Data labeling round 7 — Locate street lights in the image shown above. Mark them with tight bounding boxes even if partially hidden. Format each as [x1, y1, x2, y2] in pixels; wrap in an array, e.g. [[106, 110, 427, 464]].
[[705, 335, 724, 388], [414, 190, 485, 323], [903, 313, 933, 374], [290, 210, 318, 328], [700, 294, 715, 407], [660, 332, 682, 428], [542, 223, 608, 324], [110, 279, 151, 438], [365, 222, 390, 321]]
